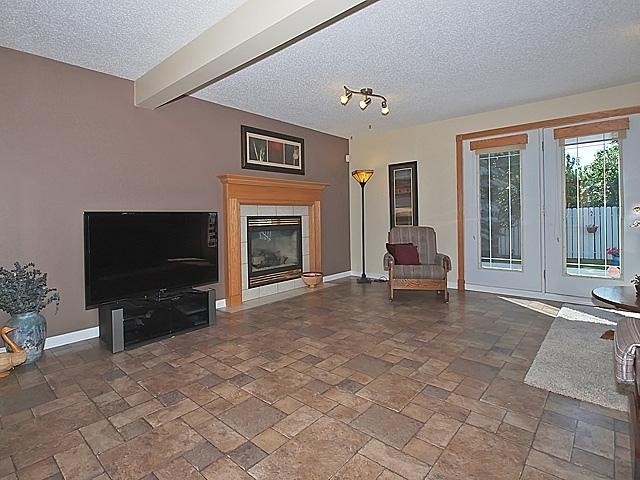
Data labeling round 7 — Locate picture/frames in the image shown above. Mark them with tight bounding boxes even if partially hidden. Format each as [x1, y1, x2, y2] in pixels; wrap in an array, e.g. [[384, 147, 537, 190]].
[[387, 161, 419, 229], [239, 125, 306, 175]]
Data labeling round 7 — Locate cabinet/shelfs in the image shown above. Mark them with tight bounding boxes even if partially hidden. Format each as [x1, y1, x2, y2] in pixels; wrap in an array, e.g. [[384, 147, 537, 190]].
[[100, 287, 217, 352]]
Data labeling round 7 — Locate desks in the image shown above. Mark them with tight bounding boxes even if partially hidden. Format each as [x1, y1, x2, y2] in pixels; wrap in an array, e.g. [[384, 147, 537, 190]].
[[591, 286, 640, 322]]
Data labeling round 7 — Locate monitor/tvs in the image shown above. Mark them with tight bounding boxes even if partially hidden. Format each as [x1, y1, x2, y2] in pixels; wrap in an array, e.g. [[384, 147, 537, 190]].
[[82, 210, 219, 311]]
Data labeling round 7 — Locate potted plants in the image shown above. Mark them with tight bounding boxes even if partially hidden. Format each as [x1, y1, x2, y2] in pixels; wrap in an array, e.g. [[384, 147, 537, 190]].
[[0, 262, 62, 367]]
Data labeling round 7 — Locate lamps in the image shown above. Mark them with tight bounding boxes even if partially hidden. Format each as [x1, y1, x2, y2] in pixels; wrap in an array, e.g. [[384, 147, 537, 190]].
[[338, 85, 389, 115], [351, 169, 375, 285]]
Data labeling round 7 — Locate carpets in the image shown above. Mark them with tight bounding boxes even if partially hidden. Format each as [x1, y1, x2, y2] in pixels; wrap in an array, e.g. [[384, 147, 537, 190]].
[[524, 302, 639, 415]]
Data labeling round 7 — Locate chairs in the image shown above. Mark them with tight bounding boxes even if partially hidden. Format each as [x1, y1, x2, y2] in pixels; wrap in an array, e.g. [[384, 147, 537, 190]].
[[384, 225, 451, 304]]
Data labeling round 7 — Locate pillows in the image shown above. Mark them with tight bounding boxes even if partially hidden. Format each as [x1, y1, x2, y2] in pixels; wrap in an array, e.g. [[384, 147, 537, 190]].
[[386, 242, 420, 265]]
